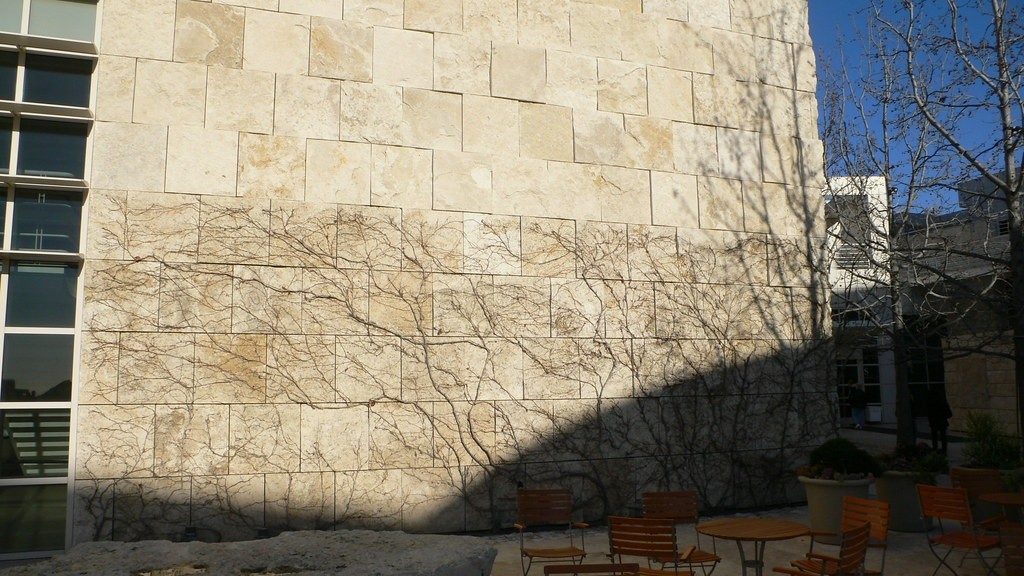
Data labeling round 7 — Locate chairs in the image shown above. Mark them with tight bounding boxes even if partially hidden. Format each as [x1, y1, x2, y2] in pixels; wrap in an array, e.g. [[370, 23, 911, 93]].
[[513, 487, 1024, 576]]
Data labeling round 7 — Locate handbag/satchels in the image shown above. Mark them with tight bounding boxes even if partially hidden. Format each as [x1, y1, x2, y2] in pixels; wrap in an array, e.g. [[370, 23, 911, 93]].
[[944, 406, 952, 418]]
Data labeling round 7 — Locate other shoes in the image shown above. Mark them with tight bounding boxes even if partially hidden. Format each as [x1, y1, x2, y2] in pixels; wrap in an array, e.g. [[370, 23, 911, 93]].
[[856, 424, 860, 428]]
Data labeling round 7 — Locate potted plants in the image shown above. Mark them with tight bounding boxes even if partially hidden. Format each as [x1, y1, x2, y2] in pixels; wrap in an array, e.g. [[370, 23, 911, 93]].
[[798, 439, 876, 544], [950, 415, 1024, 546], [871, 440, 943, 531]]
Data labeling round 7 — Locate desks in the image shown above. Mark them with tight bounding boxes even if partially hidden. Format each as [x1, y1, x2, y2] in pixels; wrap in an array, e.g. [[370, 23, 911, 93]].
[[978, 493, 1024, 506], [694, 518, 810, 576]]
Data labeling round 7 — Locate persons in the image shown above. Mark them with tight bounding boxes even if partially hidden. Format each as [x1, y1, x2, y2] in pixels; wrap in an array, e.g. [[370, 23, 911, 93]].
[[847, 380, 867, 430], [926, 391, 952, 456], [908, 393, 922, 446]]
